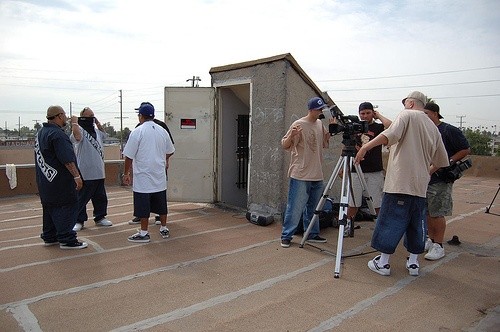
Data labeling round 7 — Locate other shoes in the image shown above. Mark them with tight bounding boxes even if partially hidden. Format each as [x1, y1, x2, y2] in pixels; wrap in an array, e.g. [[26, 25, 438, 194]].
[[129, 217, 142, 224], [342, 227, 351, 238], [281, 239, 291, 247], [307, 237, 327, 243], [155, 216, 161, 224]]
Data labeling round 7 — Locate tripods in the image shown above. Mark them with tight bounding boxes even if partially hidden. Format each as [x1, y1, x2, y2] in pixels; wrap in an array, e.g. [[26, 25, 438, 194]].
[[299, 136, 378, 278]]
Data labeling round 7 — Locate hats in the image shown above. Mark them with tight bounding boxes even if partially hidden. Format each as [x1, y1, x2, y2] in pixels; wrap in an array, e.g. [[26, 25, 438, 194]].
[[135, 102, 154, 111], [138, 105, 154, 116], [402, 91, 427, 105], [308, 97, 327, 110], [424, 102, 444, 119]]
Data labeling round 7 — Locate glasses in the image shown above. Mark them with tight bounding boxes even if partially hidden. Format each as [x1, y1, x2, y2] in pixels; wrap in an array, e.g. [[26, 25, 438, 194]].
[[45, 113, 63, 120]]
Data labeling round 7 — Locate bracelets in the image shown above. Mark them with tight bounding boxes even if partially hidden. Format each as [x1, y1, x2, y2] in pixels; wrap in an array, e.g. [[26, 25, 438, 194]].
[[74, 175, 80, 179], [72, 123, 77, 125]]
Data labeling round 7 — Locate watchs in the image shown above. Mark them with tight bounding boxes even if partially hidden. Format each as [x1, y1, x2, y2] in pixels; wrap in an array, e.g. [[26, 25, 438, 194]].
[[449, 157, 453, 165]]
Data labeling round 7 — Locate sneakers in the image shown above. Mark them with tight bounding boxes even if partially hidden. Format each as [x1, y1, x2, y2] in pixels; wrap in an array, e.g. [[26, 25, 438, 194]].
[[367, 255, 391, 276], [128, 231, 150, 242], [405, 256, 420, 275], [159, 226, 169, 238], [44, 240, 59, 245], [96, 218, 112, 226], [423, 238, 445, 260], [72, 223, 84, 232], [60, 241, 88, 250]]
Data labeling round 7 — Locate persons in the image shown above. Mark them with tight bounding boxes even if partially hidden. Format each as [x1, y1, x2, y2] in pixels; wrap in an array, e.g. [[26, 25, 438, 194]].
[[33, 105, 88, 249], [70, 107, 112, 232], [343, 102, 392, 237], [280, 98, 336, 247], [121, 102, 175, 242], [422, 103, 470, 261], [353, 92, 450, 275]]
